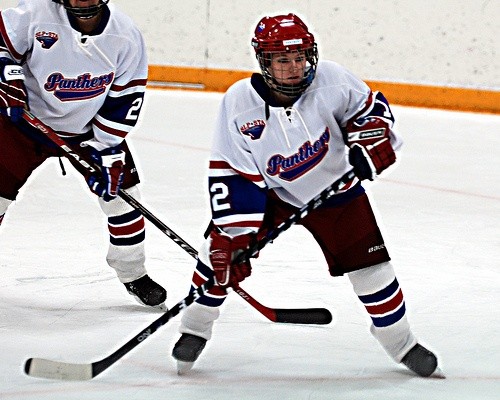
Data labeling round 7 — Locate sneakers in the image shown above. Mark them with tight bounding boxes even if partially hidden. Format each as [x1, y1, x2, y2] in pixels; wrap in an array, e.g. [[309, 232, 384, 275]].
[[171, 332, 207, 375], [401, 343, 446, 379], [123, 274, 169, 312]]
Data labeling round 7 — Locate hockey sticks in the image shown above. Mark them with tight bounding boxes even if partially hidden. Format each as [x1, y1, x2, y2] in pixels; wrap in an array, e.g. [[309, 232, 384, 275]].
[[18, 108, 332, 326], [19, 166, 356, 382]]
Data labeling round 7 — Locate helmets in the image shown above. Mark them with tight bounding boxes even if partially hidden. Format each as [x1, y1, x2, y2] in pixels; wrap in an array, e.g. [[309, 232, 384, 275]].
[[251, 13, 319, 97]]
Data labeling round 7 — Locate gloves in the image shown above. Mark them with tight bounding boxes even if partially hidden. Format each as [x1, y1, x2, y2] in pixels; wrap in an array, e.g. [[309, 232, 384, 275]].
[[348, 118, 395, 182], [0, 78, 30, 125], [85, 147, 125, 203], [208, 229, 259, 288]]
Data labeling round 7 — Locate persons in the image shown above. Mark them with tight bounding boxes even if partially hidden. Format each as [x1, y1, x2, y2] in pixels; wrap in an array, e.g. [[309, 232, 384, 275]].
[[171, 13, 438, 378], [0, 0, 167, 306]]
